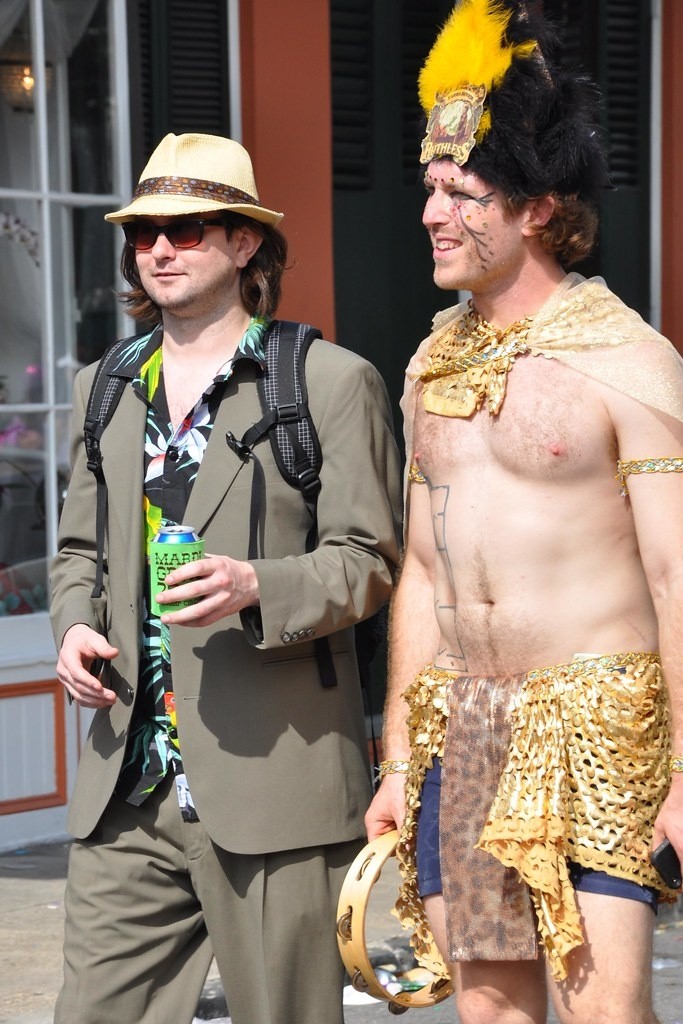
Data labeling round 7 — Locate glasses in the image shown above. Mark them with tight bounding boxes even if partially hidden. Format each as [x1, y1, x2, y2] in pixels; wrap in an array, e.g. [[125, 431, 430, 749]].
[[121, 218, 228, 249]]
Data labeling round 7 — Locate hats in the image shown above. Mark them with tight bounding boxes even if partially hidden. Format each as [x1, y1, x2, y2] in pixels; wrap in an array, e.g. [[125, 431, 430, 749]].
[[103, 132, 284, 228]]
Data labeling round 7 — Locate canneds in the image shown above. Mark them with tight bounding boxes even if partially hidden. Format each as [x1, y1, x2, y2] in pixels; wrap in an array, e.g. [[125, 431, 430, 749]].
[[153, 526, 200, 544]]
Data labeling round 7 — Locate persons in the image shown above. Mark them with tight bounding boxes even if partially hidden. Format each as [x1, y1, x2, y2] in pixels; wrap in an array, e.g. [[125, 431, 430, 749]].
[[49, 133, 402, 1024], [363, 0, 683, 1024]]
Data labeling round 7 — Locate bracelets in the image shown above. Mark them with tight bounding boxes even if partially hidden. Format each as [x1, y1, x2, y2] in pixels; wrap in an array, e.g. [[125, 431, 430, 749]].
[[378, 760, 410, 781], [669, 756, 683, 772]]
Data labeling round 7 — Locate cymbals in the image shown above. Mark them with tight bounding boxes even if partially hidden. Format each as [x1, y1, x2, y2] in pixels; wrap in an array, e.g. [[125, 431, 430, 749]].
[[333, 829, 455, 1018]]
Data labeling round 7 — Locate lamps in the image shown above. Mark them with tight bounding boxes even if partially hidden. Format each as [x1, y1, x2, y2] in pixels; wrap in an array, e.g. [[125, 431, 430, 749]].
[[0, 23, 55, 114]]
